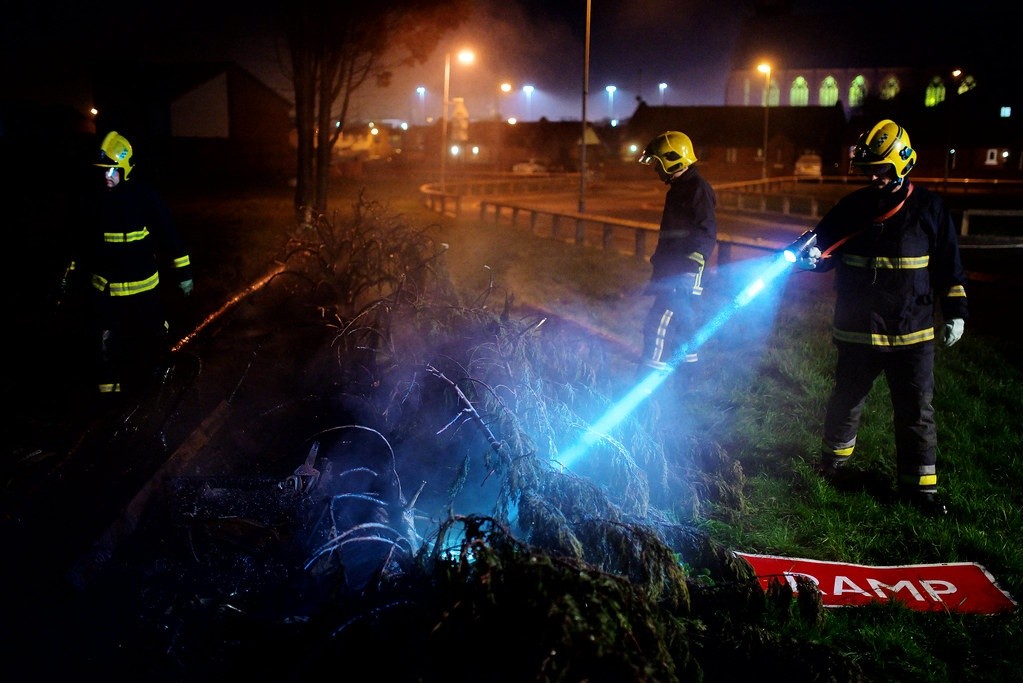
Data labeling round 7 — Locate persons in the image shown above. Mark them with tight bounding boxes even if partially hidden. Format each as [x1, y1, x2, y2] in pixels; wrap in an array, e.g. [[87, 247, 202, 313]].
[[631, 130, 725, 402], [800, 119, 969, 517], [56, 128, 193, 447]]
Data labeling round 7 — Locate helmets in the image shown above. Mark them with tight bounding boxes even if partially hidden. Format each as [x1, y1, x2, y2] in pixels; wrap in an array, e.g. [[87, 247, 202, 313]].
[[90, 131, 135, 182], [852, 119, 918, 180], [639, 131, 699, 174]]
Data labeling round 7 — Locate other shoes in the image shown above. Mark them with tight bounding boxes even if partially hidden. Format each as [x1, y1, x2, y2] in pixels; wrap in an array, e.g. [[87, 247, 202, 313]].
[[818, 455, 840, 486], [897, 483, 949, 517]]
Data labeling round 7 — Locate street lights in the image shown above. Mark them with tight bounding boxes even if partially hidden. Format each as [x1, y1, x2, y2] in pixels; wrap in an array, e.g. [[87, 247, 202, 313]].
[[438, 46, 478, 213], [755, 60, 772, 215]]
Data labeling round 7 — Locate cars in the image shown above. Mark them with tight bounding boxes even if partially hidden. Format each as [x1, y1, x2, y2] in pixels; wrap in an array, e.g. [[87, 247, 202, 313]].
[[511, 157, 546, 173], [793, 153, 823, 183], [547, 154, 580, 173]]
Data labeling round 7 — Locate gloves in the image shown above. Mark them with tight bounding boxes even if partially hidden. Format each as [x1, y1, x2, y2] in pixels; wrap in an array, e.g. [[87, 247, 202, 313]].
[[799, 247, 822, 272], [940, 318, 964, 346], [670, 272, 696, 301]]
